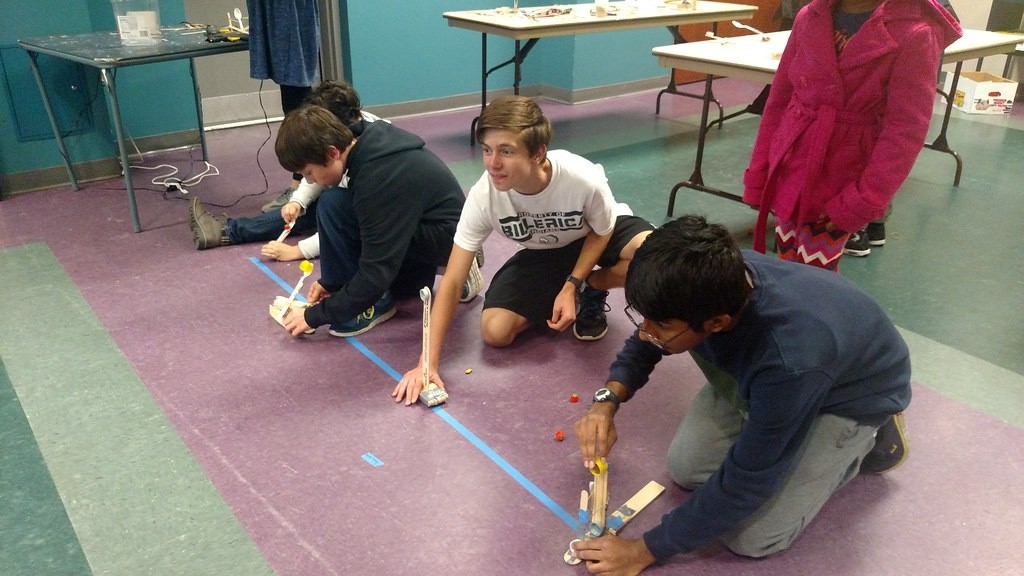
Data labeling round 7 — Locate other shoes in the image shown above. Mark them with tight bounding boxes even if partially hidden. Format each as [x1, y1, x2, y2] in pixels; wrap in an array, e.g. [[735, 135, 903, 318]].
[[191, 196, 224, 249]]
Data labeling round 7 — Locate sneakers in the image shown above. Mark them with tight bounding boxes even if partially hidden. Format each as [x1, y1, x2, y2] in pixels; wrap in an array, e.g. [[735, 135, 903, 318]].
[[572, 272, 612, 341], [863, 412, 906, 474], [261, 187, 299, 213], [866, 222, 887, 246], [327, 290, 396, 338], [844, 232, 872, 257], [458, 256, 484, 302]]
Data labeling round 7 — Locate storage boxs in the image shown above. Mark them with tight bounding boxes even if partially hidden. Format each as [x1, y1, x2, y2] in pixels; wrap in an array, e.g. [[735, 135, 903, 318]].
[[940, 68, 1017, 116]]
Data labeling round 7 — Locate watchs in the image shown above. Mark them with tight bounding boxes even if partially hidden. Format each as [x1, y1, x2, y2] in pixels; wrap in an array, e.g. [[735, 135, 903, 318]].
[[567, 276, 586, 294], [592, 388, 620, 411]]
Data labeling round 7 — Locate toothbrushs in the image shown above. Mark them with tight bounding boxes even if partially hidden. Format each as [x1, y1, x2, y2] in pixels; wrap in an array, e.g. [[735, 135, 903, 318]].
[[705, 31, 723, 40]]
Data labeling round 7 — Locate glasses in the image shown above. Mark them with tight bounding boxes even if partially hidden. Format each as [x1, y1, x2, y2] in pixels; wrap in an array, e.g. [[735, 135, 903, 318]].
[[624, 302, 706, 349]]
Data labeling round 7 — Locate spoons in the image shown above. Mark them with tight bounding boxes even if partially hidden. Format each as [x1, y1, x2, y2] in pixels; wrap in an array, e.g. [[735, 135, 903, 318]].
[[732, 20, 763, 34], [234, 7, 244, 30]]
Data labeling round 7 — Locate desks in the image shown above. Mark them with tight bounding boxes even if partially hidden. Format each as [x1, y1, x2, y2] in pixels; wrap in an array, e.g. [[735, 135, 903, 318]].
[[20, 26, 256, 234], [442, 0, 758, 148], [652, 25, 1024, 254]]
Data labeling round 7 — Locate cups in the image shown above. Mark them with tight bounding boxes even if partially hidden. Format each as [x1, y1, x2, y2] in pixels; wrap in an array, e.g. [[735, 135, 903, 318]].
[[595, 0, 609, 17]]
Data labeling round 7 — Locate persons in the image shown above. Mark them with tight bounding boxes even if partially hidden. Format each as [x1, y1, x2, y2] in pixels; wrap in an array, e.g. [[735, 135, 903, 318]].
[[741, 0, 958, 275], [274, 104, 484, 337], [188, 80, 391, 261], [575, 216, 912, 575], [843, 200, 893, 257], [392, 96, 654, 406], [246, 0, 320, 214]]
